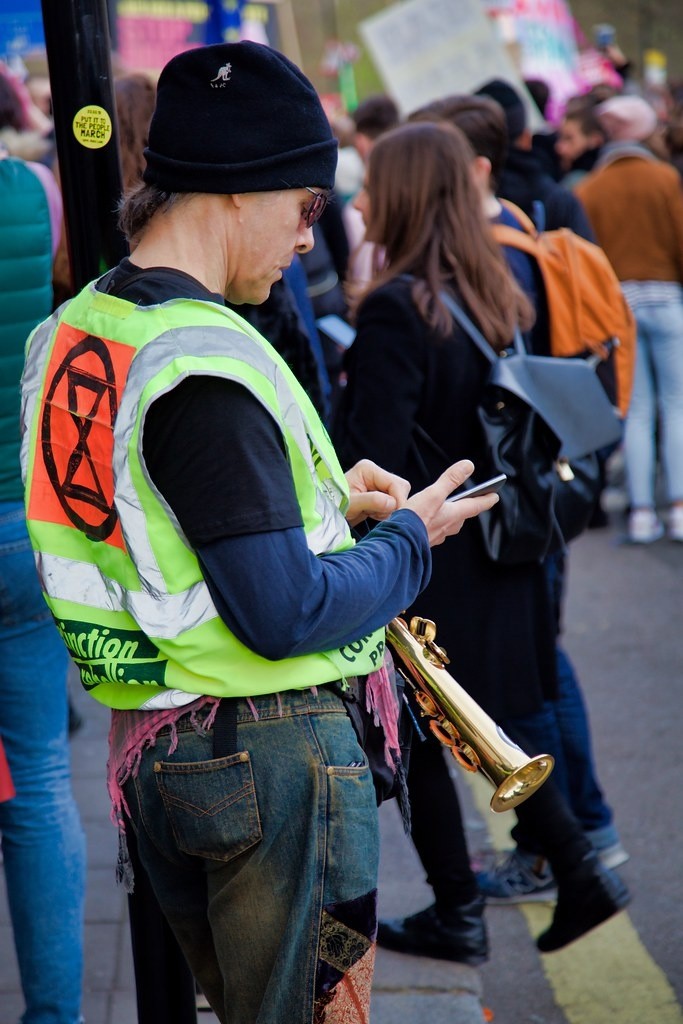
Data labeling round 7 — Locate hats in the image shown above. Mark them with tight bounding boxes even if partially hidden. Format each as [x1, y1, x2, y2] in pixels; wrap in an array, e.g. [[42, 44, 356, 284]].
[[478, 81, 527, 142], [139, 41, 338, 194], [596, 93, 657, 143]]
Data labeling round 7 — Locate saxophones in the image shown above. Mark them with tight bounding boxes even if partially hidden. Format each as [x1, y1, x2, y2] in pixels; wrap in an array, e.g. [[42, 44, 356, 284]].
[[385, 615, 561, 816]]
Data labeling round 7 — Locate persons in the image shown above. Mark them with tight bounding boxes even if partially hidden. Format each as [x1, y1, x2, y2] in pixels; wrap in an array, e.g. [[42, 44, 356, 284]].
[[0, 26, 156, 1024], [231, 22, 683, 965], [16, 40, 499, 1024]]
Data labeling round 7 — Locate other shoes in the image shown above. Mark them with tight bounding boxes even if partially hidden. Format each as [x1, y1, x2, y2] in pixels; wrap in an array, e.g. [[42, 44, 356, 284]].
[[665, 508, 683, 542], [629, 511, 664, 544]]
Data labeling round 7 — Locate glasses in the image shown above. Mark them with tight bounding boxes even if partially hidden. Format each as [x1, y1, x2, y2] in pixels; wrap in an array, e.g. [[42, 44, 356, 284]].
[[303, 185, 329, 228]]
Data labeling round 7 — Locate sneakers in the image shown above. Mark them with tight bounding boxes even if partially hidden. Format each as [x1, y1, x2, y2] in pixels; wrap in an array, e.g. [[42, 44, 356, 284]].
[[582, 822, 630, 869], [474, 851, 560, 905]]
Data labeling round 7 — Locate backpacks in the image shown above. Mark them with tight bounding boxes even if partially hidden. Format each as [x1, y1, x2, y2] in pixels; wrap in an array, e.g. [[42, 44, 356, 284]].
[[484, 199, 635, 420], [398, 275, 625, 565]]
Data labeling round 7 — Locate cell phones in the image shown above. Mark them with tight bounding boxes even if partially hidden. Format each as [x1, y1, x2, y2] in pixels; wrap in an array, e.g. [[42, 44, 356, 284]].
[[314, 311, 358, 349], [447, 472, 507, 502]]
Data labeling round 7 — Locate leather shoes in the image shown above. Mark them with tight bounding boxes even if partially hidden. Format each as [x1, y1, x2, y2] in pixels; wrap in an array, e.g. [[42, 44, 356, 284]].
[[375, 896, 489, 966], [537, 871, 631, 953]]
